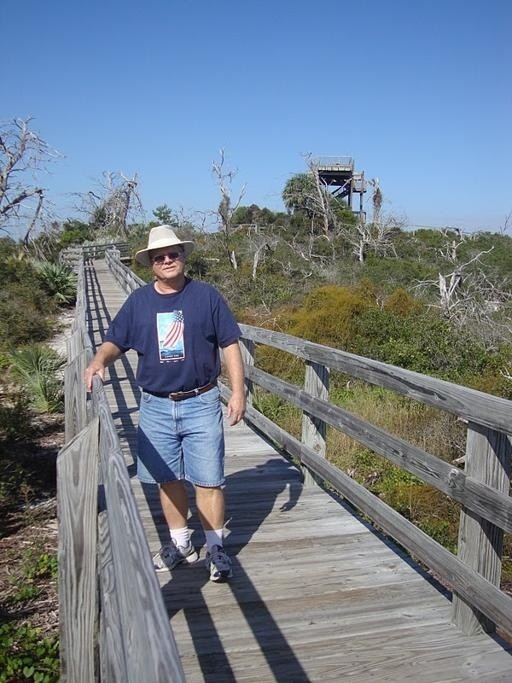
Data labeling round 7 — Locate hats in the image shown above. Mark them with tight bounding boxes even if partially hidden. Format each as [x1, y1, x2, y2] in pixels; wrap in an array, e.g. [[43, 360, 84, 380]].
[[135, 224, 195, 266]]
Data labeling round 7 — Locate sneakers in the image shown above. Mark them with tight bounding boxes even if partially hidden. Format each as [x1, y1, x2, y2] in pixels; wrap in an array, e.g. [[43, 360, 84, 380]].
[[205, 544, 233, 583], [152, 539, 198, 572]]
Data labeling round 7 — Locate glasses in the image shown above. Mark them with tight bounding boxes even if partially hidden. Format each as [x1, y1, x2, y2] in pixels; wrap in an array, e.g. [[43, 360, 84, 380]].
[[153, 251, 180, 265]]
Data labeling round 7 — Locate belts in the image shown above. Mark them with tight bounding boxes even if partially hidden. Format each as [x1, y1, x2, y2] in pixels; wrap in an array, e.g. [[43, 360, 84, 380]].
[[143, 382, 215, 401]]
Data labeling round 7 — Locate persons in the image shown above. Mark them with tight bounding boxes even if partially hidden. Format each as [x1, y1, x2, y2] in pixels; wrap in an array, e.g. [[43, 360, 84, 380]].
[[84, 224, 246, 581]]
